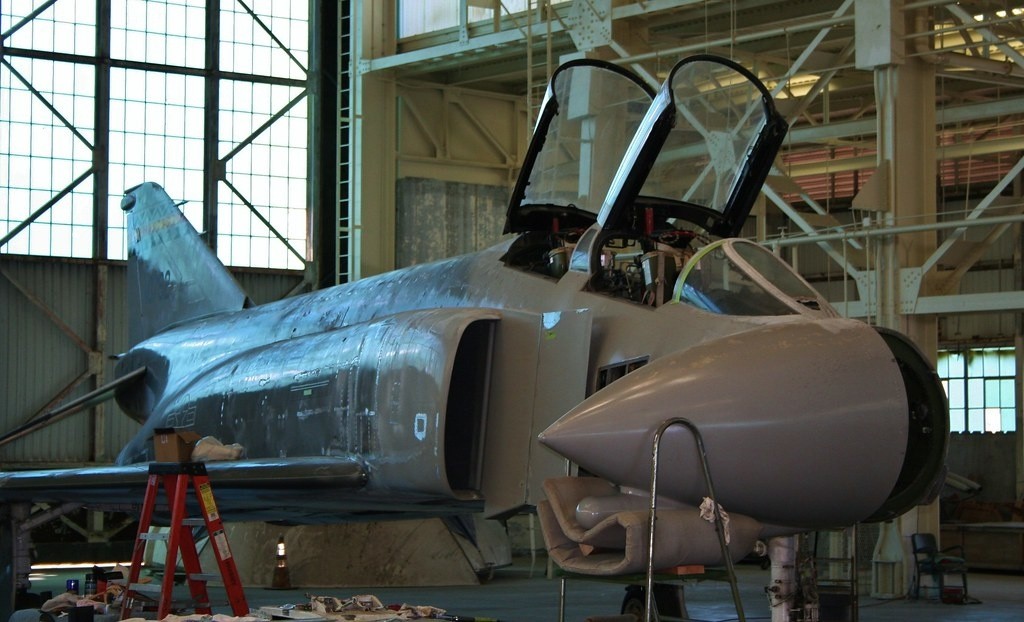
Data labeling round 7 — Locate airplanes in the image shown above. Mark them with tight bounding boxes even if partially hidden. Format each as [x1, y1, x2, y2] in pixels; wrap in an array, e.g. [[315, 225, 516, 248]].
[[1, 52, 994, 542]]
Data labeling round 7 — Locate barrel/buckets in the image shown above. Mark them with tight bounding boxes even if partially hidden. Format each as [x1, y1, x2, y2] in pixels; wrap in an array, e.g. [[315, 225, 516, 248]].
[[66, 580, 79, 596]]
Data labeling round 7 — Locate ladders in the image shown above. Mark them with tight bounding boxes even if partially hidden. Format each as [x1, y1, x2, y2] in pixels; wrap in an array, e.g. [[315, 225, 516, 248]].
[[117, 461, 250, 622]]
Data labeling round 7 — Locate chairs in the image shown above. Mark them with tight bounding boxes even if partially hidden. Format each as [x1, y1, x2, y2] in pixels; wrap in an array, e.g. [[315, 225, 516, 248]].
[[911, 533, 968, 602]]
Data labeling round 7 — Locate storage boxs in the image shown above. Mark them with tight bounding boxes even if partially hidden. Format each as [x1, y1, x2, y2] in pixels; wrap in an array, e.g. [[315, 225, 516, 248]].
[[153, 427, 201, 463]]
